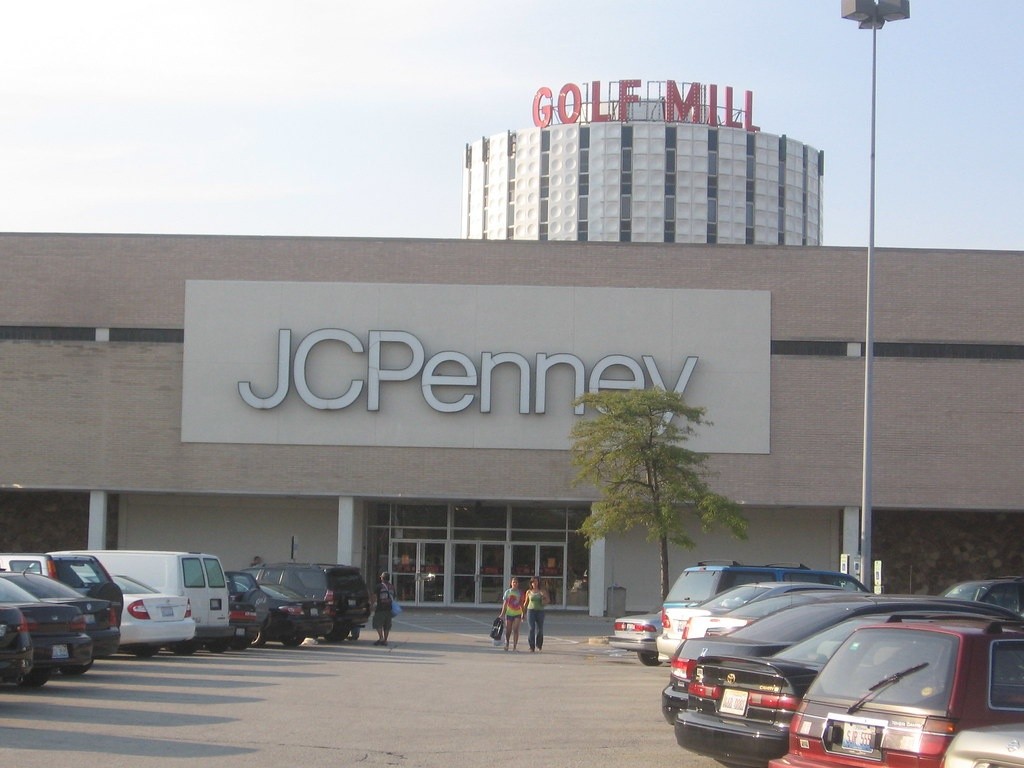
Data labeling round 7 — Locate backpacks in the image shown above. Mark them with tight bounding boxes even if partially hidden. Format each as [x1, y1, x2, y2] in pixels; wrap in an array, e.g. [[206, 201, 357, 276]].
[[377, 582, 392, 611]]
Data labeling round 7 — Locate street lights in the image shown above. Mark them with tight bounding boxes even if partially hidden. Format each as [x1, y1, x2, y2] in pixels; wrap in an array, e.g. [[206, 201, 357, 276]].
[[841, 1, 911, 599]]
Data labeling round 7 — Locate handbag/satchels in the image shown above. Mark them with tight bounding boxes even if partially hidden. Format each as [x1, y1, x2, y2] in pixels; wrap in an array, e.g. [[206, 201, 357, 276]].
[[489, 617, 505, 646], [391, 596, 402, 617]]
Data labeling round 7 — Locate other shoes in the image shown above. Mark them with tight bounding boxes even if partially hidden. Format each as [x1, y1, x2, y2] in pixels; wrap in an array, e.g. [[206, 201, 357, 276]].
[[374, 639, 388, 646], [537, 647, 543, 653], [512, 648, 519, 652], [503, 645, 509, 651], [528, 647, 535, 652]]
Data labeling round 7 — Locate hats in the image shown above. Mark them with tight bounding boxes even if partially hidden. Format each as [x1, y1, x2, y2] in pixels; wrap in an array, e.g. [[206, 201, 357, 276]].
[[379, 571, 390, 580]]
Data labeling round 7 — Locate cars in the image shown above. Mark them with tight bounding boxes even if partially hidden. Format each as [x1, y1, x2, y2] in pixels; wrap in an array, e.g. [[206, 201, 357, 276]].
[[939, 721, 1024, 768], [0, 606, 35, 681], [606, 609, 662, 668], [248, 580, 334, 647], [0, 578, 94, 688], [939, 577, 1024, 618], [655, 581, 846, 662], [767, 614, 1024, 768], [675, 611, 1010, 768], [678, 591, 862, 652], [0, 570, 120, 676], [77, 573, 196, 658], [660, 596, 1012, 726]]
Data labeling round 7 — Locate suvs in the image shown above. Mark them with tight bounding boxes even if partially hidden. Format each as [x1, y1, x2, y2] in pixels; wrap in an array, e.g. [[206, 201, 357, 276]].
[[224, 570, 270, 650], [240, 562, 372, 640], [656, 558, 871, 663], [0, 552, 124, 655]]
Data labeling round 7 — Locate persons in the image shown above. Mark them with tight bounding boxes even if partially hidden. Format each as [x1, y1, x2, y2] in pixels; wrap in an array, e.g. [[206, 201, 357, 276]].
[[368, 572, 395, 646], [498, 577, 525, 654], [523, 577, 550, 652], [250, 556, 261, 567]]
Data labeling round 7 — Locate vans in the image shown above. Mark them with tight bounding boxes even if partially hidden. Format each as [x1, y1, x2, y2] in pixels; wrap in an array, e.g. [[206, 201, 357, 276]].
[[46, 551, 230, 655]]
